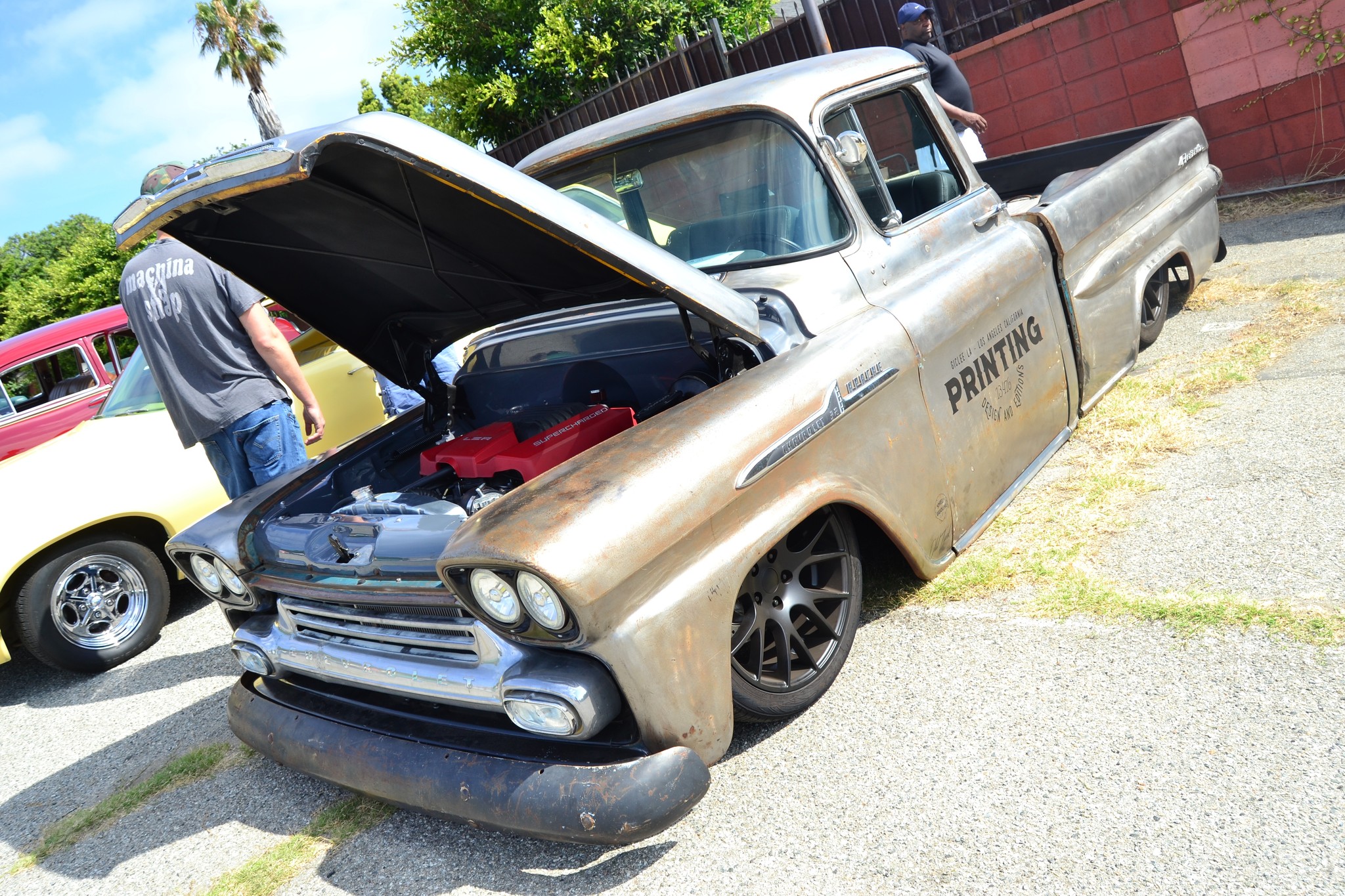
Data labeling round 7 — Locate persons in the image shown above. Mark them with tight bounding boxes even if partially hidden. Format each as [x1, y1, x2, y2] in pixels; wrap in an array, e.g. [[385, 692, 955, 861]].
[[118, 164, 326, 501], [372, 342, 462, 418], [897, 2, 988, 174]]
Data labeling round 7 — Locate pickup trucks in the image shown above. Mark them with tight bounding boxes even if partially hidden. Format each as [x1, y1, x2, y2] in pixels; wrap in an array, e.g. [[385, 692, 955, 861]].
[[110, 45, 1231, 852]]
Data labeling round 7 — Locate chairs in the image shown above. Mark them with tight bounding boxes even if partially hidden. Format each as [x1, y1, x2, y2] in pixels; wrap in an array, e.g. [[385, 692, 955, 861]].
[[49, 372, 115, 401], [666, 206, 801, 257], [796, 171, 959, 254]]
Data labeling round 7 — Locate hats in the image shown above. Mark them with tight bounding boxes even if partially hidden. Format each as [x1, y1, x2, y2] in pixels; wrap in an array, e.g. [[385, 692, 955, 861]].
[[141, 161, 190, 196], [898, 3, 935, 26]]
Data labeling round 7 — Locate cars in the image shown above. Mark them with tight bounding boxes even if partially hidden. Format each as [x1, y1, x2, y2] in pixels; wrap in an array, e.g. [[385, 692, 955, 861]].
[[0, 295, 310, 464], [0, 323, 396, 676]]
